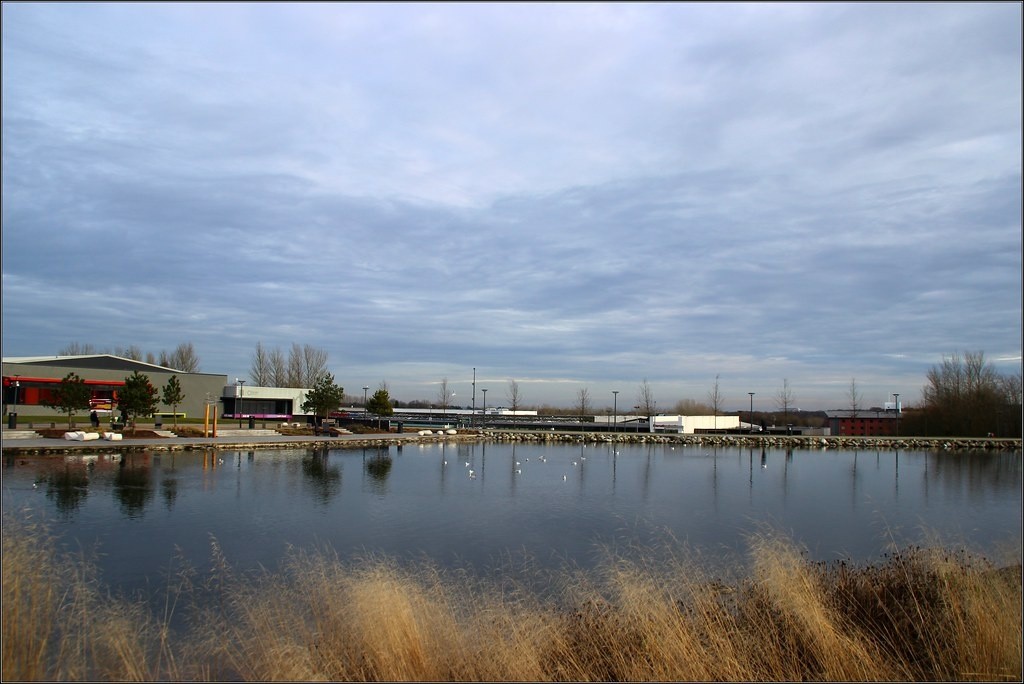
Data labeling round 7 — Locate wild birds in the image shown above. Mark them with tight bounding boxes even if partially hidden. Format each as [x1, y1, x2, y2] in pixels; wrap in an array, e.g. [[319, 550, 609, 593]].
[[443, 443, 768, 482], [452, 389, 456, 396]]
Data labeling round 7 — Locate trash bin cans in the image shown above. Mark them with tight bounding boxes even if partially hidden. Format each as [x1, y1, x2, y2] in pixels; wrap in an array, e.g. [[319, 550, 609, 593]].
[[398, 446, 402, 451], [398, 421, 403, 433], [786, 424, 793, 436], [249, 416, 255, 429], [9, 413, 17, 429]]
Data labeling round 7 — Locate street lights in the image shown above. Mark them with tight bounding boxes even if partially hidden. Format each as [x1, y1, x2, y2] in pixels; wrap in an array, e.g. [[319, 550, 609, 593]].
[[238, 380, 246, 417], [892, 393, 900, 437], [362, 387, 370, 422], [748, 392, 756, 434], [482, 388, 488, 429], [612, 391, 619, 432]]
